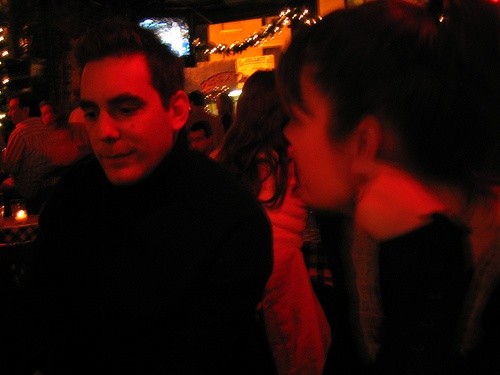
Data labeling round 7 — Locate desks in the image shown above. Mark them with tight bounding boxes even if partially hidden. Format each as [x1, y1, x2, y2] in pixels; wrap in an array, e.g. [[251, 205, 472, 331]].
[[0, 214, 40, 253]]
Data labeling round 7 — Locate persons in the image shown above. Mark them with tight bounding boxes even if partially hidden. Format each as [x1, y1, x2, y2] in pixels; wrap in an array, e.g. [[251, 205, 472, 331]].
[[0, 0, 500, 374]]
